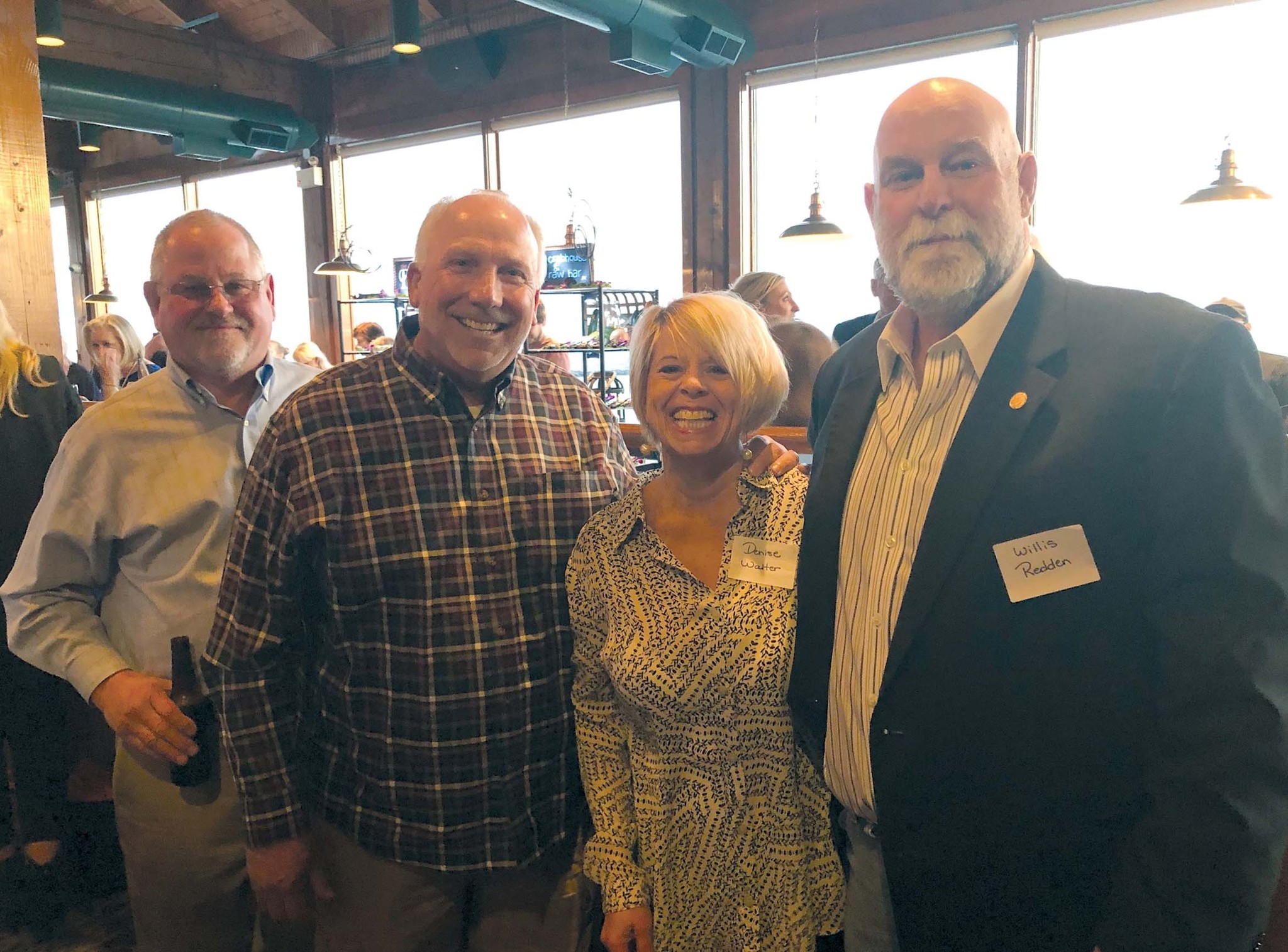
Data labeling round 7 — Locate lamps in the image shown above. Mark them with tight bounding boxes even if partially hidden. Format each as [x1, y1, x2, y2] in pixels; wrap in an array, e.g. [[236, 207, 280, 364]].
[[313, 58, 365, 275], [780, 0, 843, 237], [35, 0, 65, 46], [78, 126, 100, 151], [82, 160, 119, 303], [1180, 136, 1273, 205], [293, 156, 323, 189], [390, 0, 421, 53]]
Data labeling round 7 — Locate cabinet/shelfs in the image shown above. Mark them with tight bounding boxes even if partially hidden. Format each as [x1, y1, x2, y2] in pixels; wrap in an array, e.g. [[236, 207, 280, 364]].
[[338, 297, 418, 361], [525, 283, 662, 475]]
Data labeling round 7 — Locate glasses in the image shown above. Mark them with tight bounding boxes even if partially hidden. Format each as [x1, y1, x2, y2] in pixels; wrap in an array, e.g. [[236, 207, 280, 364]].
[[152, 279, 263, 301]]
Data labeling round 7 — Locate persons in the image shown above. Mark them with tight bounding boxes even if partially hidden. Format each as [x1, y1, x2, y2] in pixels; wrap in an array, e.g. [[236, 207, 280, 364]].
[[197, 192, 810, 952], [0, 208, 324, 952], [522, 300, 569, 372], [564, 291, 844, 952], [292, 342, 333, 371], [732, 255, 900, 428], [787, 77, 1288, 952], [353, 321, 394, 357], [1203, 298, 1288, 431]]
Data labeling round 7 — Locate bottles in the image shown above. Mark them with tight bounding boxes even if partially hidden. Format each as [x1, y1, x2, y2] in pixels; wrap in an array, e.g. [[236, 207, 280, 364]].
[[166, 635, 220, 786]]
